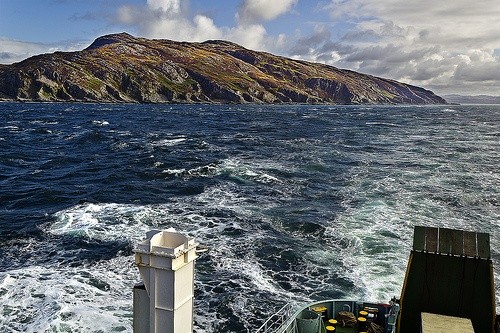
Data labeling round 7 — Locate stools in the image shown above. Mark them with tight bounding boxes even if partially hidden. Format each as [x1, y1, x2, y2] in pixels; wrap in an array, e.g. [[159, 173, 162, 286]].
[[325, 326, 336, 333], [329, 319, 337, 326], [355, 317, 366, 333], [358, 311, 370, 318]]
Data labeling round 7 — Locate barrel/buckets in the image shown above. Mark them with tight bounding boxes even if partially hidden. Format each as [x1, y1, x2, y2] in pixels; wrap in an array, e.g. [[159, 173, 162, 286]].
[[296, 309, 319, 333]]
